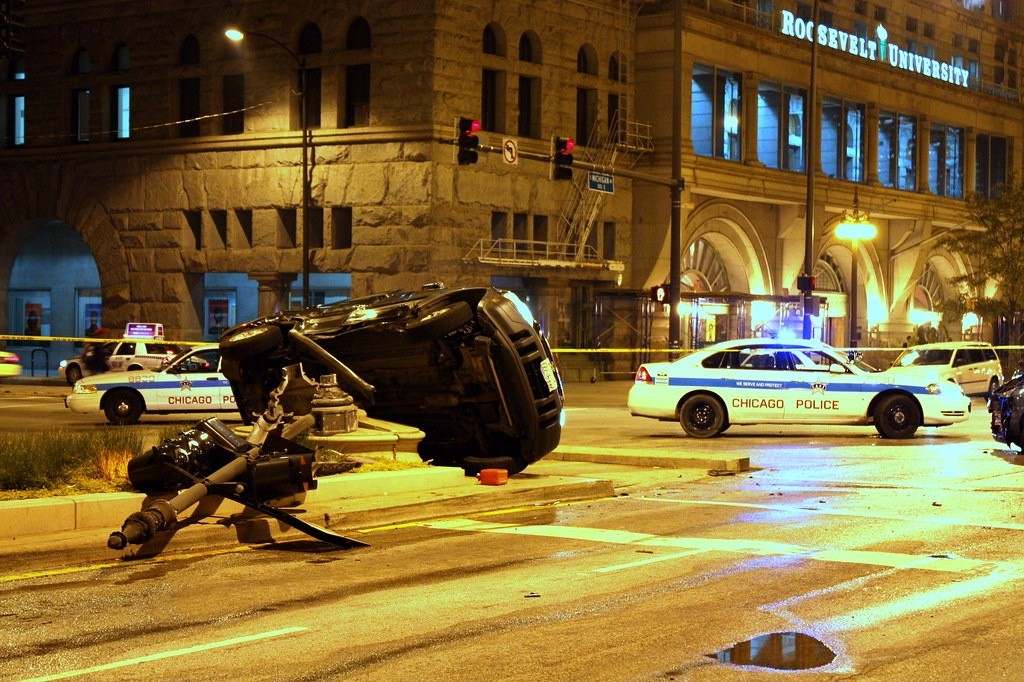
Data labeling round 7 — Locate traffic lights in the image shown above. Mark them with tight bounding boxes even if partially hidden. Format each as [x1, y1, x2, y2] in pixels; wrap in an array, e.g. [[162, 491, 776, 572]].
[[457, 118, 480, 165], [650, 285, 668, 304], [797, 275, 816, 292], [549, 134, 575, 180]]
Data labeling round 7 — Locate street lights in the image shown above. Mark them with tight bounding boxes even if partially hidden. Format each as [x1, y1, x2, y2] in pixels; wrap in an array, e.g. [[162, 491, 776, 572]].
[[219, 28, 313, 313], [833, 186, 877, 349]]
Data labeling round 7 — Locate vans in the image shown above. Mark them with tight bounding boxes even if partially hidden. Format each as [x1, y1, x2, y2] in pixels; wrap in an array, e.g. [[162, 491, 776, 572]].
[[888, 341, 1005, 402]]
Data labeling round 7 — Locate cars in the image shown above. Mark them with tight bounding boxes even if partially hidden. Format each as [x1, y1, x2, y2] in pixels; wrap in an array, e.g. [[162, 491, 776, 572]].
[[985, 375, 1023, 456], [0, 350, 24, 380], [218, 282, 565, 477], [63, 343, 239, 425], [628, 338, 970, 439], [57, 321, 210, 389]]
